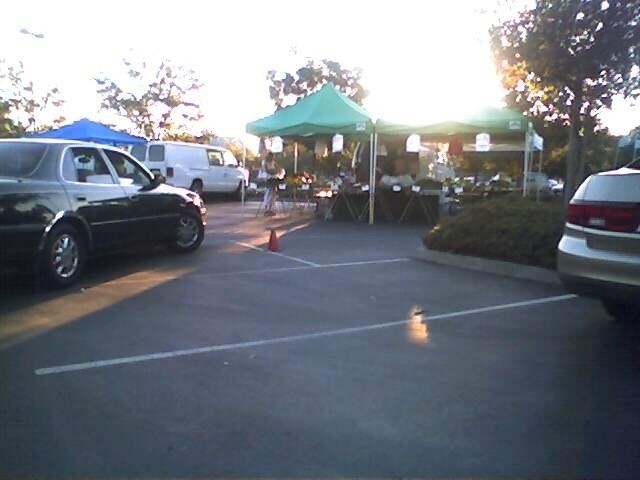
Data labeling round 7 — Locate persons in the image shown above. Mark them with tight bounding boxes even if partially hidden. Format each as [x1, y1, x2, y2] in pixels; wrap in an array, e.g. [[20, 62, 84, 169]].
[[260, 151, 282, 217]]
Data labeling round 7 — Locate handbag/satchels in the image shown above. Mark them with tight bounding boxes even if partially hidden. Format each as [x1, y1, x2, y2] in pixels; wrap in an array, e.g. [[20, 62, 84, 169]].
[[257, 167, 267, 184]]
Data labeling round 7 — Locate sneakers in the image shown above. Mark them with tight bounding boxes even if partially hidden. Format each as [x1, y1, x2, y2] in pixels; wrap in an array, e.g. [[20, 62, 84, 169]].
[[264, 211, 277, 216]]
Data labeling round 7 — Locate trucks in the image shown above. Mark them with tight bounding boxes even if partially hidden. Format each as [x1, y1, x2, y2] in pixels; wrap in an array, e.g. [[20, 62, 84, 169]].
[[133, 140, 251, 203]]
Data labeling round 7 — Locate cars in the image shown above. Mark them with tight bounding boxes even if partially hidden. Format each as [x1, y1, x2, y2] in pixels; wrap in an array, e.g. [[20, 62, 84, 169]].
[[555, 153, 639, 324], [410, 170, 564, 195], [1, 134, 209, 287]]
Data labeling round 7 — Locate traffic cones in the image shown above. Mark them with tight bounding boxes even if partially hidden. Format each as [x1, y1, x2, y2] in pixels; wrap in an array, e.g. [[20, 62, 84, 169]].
[[266, 229, 280, 252]]
